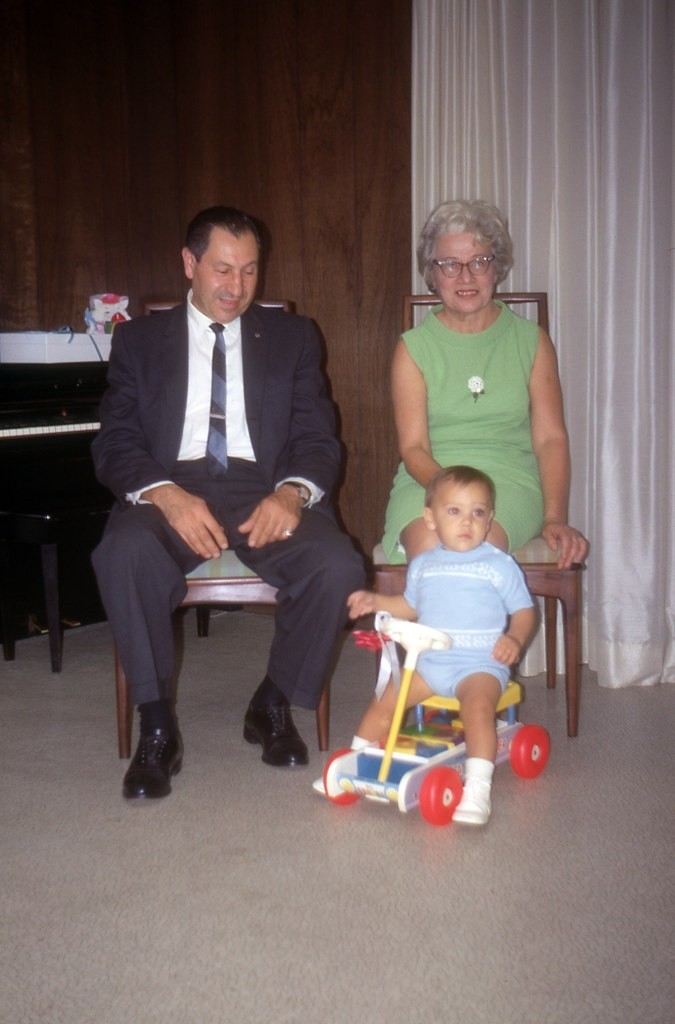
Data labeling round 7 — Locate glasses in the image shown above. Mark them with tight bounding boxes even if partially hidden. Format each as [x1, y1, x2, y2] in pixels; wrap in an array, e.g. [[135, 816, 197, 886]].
[[432, 254, 496, 278]]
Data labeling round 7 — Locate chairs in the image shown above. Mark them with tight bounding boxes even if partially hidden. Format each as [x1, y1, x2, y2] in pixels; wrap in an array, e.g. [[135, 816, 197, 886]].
[[372, 293, 585, 738], [114, 297, 329, 759]]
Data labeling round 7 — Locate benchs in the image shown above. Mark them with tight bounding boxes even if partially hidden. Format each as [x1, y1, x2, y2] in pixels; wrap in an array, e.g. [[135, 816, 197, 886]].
[[11, 499, 211, 676]]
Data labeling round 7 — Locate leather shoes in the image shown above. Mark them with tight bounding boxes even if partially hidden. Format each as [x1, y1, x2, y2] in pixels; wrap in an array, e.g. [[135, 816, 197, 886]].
[[243, 701, 310, 770], [123, 725, 184, 800]]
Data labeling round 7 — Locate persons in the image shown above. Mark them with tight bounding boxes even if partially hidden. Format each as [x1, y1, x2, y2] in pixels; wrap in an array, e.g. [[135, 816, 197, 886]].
[[311, 465, 534, 825], [92, 207, 368, 799], [381, 200, 591, 569]]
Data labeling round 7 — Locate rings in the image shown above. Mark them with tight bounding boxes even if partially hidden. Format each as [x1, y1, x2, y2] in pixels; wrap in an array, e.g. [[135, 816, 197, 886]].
[[285, 529, 292, 538], [572, 538, 576, 542]]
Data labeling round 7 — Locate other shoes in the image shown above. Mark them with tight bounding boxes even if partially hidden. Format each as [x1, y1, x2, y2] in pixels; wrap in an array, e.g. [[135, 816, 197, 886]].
[[451, 796, 491, 824], [312, 777, 325, 793]]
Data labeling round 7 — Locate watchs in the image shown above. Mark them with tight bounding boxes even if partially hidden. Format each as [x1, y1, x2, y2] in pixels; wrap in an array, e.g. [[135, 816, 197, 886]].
[[282, 482, 310, 505]]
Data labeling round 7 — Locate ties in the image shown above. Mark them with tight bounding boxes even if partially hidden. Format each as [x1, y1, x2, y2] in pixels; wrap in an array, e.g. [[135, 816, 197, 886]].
[[206, 322, 229, 481]]
